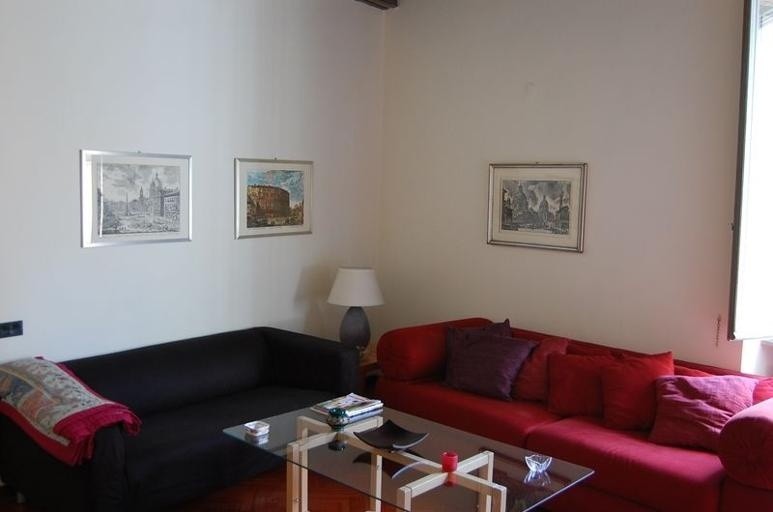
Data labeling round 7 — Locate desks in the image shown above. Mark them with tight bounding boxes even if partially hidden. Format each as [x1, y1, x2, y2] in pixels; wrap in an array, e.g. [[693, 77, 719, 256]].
[[221, 392, 597, 510]]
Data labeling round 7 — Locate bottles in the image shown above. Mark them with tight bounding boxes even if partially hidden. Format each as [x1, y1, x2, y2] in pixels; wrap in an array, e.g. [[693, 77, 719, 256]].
[[327, 407, 349, 432]]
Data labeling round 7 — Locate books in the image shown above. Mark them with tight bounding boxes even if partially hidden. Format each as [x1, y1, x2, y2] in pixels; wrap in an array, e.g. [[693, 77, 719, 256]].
[[309, 392, 384, 423]]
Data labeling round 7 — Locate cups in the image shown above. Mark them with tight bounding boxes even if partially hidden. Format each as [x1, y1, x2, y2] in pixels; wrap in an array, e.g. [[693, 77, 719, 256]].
[[440, 452, 458, 472], [525, 454, 553, 473]]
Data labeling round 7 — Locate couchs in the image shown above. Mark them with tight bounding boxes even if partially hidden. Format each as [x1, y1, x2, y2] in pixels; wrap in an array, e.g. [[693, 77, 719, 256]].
[[372, 317, 773, 512], [0, 324, 362, 509]]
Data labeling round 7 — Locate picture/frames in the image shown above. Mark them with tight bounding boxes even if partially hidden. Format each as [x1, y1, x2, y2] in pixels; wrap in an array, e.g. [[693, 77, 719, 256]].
[[485, 161, 589, 252], [78, 149, 314, 250]]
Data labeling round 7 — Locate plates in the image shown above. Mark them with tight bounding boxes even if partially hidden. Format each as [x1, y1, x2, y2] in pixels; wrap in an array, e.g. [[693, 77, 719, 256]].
[[353, 420, 429, 451], [352, 446, 428, 479]]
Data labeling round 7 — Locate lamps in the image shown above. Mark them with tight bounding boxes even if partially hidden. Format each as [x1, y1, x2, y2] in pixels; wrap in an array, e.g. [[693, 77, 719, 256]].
[[325, 268, 384, 358]]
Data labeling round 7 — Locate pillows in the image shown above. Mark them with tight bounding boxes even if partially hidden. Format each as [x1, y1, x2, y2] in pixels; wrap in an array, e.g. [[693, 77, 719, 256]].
[[518, 329, 568, 402], [545, 349, 604, 420], [600, 351, 674, 430], [649, 373, 759, 449], [446, 322, 535, 404], [444, 319, 514, 355]]
